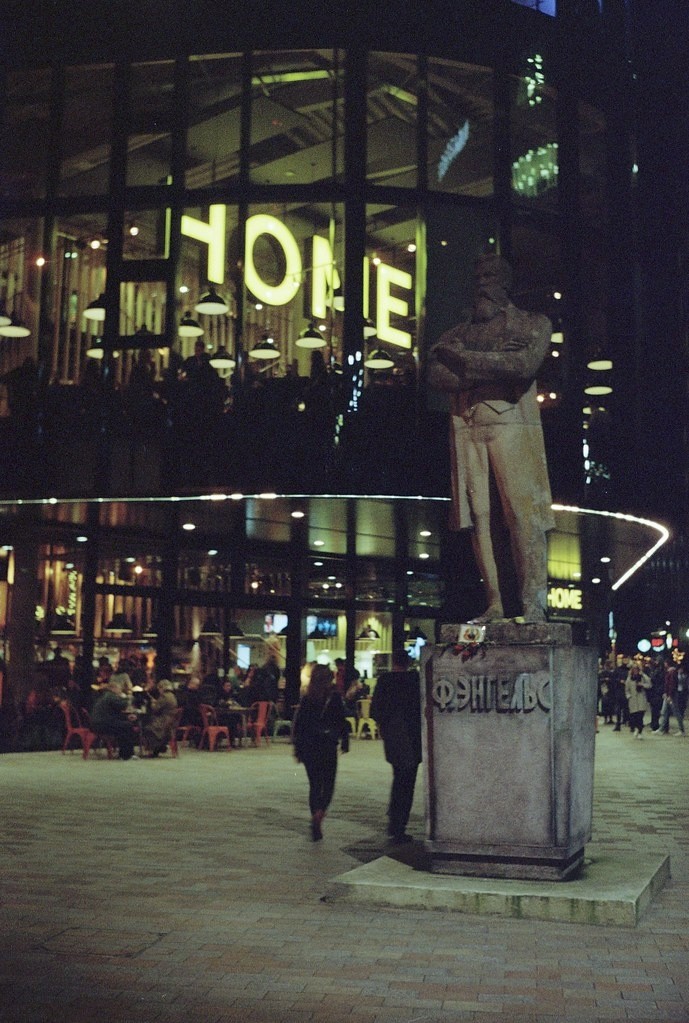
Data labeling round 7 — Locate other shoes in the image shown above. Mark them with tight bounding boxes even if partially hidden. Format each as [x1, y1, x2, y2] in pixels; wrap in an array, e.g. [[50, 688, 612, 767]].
[[388, 833, 412, 847], [673, 728, 686, 737], [652, 729, 664, 736], [633, 727, 642, 740], [308, 809, 325, 826]]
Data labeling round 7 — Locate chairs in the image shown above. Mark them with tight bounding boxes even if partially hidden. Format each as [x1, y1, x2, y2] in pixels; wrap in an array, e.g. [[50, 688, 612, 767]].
[[56, 699, 377, 760]]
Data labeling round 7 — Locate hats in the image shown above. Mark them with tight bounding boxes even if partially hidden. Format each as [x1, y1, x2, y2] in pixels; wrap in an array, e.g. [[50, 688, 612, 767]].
[[159, 679, 174, 691]]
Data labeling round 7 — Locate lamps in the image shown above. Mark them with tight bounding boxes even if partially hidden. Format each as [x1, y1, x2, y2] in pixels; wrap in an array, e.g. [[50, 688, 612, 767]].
[[80, 290, 108, 323], [49, 613, 78, 636], [227, 621, 245, 640], [248, 332, 281, 359], [106, 614, 133, 633], [355, 623, 381, 643], [208, 345, 236, 369], [363, 316, 376, 340], [408, 621, 429, 644], [295, 321, 328, 350], [200, 616, 221, 636], [277, 623, 290, 638], [83, 337, 120, 359], [141, 626, 158, 639], [363, 346, 394, 370], [306, 626, 328, 640], [0, 296, 31, 338], [176, 312, 203, 337], [194, 286, 229, 316]]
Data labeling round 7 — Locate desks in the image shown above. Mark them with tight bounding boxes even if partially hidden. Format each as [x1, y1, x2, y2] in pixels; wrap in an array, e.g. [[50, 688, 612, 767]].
[[121, 708, 152, 756], [218, 705, 260, 751]]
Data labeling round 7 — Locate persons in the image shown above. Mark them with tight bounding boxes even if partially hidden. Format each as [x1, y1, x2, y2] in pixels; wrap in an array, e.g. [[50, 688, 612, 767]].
[[593, 646, 689, 739], [0, 336, 419, 415], [368, 650, 422, 848], [426, 237, 558, 623], [0, 636, 398, 759], [293, 664, 351, 840]]
